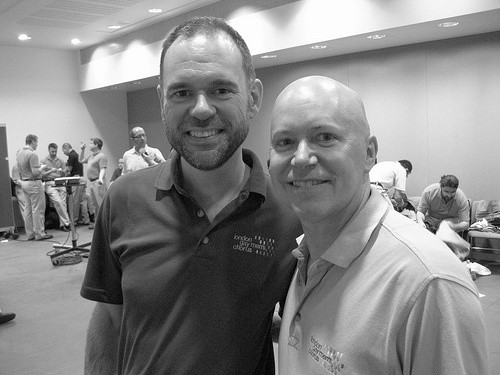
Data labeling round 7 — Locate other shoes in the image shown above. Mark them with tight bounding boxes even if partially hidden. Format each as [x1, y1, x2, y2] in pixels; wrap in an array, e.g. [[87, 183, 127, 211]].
[[61, 226, 68, 232], [0, 312, 16, 324], [36, 235, 53, 241], [67, 225, 71, 231], [28, 232, 47, 240], [88, 225, 94, 229]]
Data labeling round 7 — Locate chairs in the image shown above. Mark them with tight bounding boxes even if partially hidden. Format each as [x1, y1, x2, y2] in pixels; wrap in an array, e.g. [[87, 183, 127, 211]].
[[406, 196, 500, 265]]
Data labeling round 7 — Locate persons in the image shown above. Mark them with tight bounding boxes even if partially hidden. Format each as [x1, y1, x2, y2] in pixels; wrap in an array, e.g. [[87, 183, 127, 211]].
[[12, 150, 28, 220], [62, 143, 84, 225], [16, 134, 53, 240], [78, 137, 107, 229], [121, 126, 166, 176], [270, 75, 488, 375], [80, 16, 304, 375], [40, 143, 72, 231], [417, 174, 469, 232], [110, 158, 123, 182], [369, 160, 412, 211]]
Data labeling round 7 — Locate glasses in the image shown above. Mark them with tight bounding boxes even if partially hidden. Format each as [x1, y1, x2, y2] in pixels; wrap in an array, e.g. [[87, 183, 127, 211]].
[[134, 134, 146, 138]]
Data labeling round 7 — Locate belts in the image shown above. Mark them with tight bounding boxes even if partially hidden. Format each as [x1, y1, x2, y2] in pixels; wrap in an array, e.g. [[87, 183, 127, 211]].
[[46, 179, 54, 182], [23, 178, 41, 181], [90, 178, 99, 182], [370, 182, 383, 187]]
